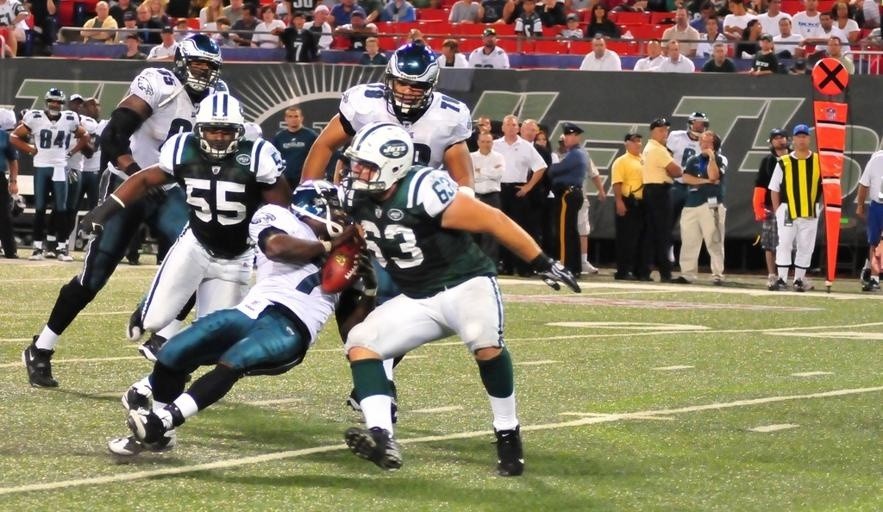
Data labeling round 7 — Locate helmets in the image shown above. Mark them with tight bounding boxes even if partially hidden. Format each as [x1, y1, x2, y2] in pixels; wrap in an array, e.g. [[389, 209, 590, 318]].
[[340, 122, 415, 210], [288, 179, 339, 220], [174, 34, 224, 92], [191, 92, 245, 163], [384, 42, 440, 120], [687, 112, 709, 138], [44, 87, 66, 116]]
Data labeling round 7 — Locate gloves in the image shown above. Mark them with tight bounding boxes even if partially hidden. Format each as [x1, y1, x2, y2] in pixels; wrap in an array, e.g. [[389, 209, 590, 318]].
[[125, 162, 168, 205], [75, 193, 126, 240], [528, 251, 581, 293]]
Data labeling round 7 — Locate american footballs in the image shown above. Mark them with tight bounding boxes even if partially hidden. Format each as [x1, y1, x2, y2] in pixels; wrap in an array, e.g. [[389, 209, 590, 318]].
[[320, 240, 360, 293]]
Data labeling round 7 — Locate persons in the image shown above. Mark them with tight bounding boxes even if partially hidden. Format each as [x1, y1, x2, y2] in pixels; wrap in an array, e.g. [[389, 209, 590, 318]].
[[611, 111, 729, 286], [0, 0, 883, 77], [104, 179, 360, 461], [270, 105, 319, 190], [18, 34, 234, 390], [331, 120, 583, 481], [298, 40, 479, 423], [468, 114, 607, 276], [855, 149, 883, 292], [753, 123, 822, 293], [75, 89, 298, 410], [0, 87, 169, 266]]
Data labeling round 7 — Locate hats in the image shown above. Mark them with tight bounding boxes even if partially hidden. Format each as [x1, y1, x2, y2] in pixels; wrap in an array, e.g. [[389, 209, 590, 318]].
[[758, 33, 773, 42], [562, 122, 584, 136], [624, 132, 642, 142], [351, 9, 365, 19], [484, 28, 496, 36], [566, 13, 579, 21], [122, 11, 187, 41], [650, 118, 671, 130], [765, 124, 810, 143], [314, 5, 329, 12], [290, 12, 305, 26], [68, 94, 84, 102]]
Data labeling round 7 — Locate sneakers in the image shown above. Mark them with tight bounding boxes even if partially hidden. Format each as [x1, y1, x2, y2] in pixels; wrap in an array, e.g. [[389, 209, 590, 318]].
[[614, 271, 693, 283], [344, 388, 402, 472], [493, 424, 524, 477], [29, 240, 73, 261], [21, 335, 58, 387], [43, 46, 51, 53], [125, 307, 168, 363], [860, 268, 880, 292], [713, 279, 722, 286], [581, 261, 599, 272], [107, 375, 177, 457], [766, 274, 815, 292]]
